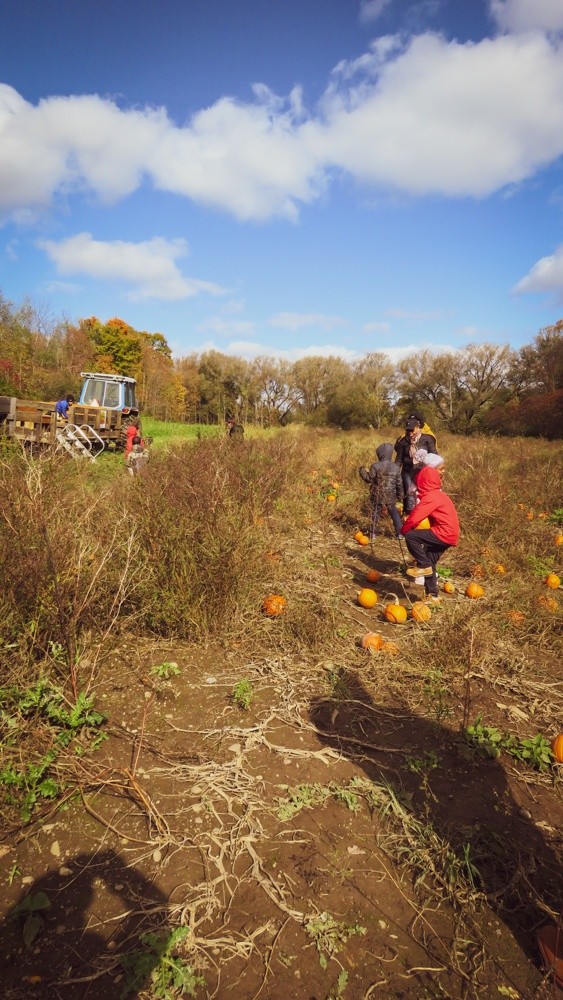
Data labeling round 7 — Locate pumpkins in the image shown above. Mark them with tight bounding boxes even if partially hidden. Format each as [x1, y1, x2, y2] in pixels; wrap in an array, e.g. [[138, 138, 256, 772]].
[[444, 498, 563, 623], [263, 594, 288, 617], [366, 568, 381, 582], [355, 588, 377, 609], [384, 598, 407, 624], [361, 633, 383, 650], [327, 482, 430, 544], [412, 603, 431, 622], [549, 732, 563, 764]]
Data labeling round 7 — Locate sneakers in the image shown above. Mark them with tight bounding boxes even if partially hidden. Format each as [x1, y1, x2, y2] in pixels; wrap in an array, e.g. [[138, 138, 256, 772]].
[[406, 566, 433, 577], [423, 597, 440, 607]]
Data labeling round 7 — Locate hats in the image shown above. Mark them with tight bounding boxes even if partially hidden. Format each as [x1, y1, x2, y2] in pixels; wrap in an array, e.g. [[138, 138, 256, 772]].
[[405, 419, 418, 428], [66, 394, 74, 402], [415, 448, 444, 468]]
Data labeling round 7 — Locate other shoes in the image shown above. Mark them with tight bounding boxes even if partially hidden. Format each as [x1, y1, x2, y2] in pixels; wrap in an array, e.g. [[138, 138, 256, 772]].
[[395, 535, 402, 539], [368, 534, 376, 539]]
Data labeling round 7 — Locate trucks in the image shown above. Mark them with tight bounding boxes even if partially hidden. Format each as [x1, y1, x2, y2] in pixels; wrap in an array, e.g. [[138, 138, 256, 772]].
[[0, 371, 142, 453]]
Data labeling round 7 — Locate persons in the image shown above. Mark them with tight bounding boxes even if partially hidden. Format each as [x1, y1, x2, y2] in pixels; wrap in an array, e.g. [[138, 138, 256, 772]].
[[124, 426, 148, 475], [359, 415, 459, 604], [227, 414, 245, 441], [55, 394, 74, 420]]
